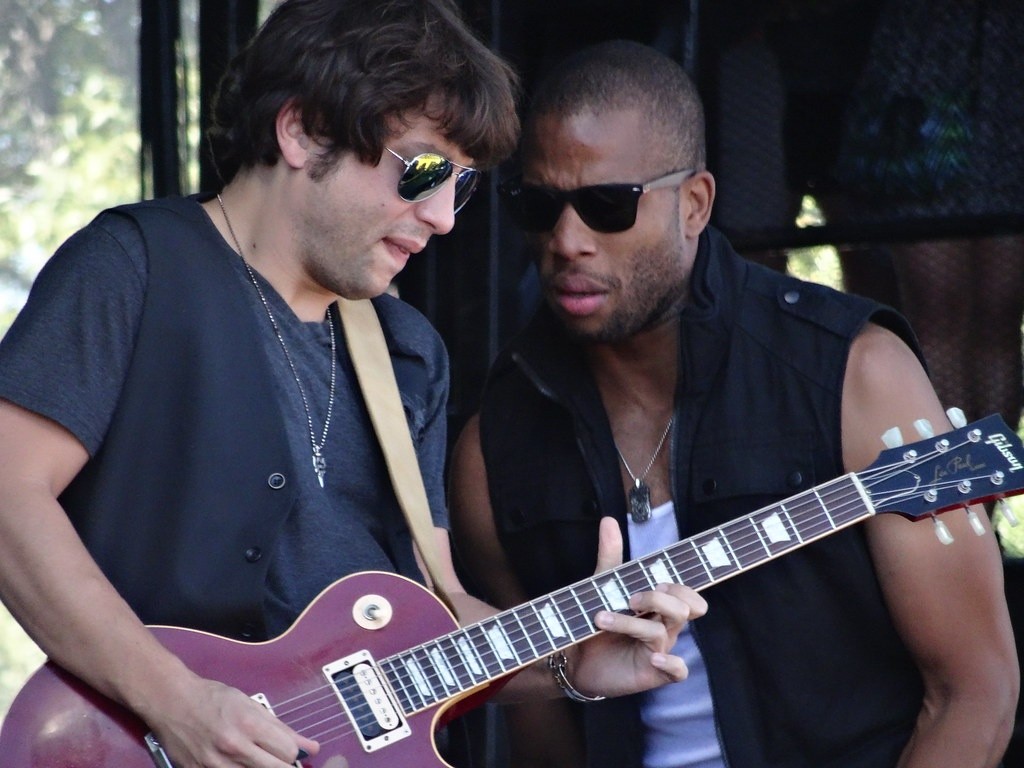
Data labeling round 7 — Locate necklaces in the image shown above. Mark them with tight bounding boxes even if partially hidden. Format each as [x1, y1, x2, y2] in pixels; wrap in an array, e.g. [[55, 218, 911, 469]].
[[217, 193, 337, 491], [615, 419, 673, 524]]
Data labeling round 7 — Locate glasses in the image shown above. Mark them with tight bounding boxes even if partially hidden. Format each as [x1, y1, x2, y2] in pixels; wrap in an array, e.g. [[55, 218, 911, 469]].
[[495, 167, 698, 234], [384, 146, 482, 215]]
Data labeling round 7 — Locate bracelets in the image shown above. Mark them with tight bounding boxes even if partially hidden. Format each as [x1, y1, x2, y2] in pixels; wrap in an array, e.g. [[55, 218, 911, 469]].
[[548, 651, 606, 703]]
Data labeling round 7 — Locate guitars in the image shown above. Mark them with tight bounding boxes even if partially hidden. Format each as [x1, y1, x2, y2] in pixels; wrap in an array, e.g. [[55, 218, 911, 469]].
[[0, 407, 1023, 768]]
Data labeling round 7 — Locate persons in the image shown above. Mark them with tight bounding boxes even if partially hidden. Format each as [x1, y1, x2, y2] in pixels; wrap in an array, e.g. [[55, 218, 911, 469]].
[[446, 37, 1021, 768], [0, 0, 708, 768]]
